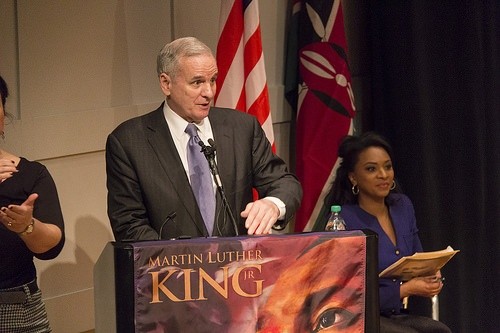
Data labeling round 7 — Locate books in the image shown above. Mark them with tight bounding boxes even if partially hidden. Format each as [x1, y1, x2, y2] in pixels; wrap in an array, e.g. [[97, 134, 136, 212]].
[[378, 246, 461, 280]]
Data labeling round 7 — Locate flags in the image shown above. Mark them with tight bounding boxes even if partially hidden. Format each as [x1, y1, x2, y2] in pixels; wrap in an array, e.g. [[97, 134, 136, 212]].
[[281, 0, 356, 236], [216, 0, 276, 202]]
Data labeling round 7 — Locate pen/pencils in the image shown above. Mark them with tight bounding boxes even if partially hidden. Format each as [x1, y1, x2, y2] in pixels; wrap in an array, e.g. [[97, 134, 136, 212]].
[[437, 278, 445, 282]]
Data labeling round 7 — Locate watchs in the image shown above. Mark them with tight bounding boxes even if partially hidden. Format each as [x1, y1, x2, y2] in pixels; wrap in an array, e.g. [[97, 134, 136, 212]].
[[17, 218, 35, 236]]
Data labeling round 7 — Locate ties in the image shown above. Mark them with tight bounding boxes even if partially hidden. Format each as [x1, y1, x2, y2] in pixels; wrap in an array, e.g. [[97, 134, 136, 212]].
[[184, 123, 216, 237]]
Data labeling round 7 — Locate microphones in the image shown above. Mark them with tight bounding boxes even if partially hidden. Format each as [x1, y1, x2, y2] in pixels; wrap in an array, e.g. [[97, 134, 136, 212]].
[[199, 139, 217, 161], [159, 212, 177, 240]]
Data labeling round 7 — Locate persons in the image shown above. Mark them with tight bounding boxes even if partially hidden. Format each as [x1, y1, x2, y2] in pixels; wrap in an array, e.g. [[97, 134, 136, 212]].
[[322, 135, 453, 333], [399, 259, 440, 280], [0, 75, 65, 333], [105, 37, 303, 242], [254, 232, 363, 333]]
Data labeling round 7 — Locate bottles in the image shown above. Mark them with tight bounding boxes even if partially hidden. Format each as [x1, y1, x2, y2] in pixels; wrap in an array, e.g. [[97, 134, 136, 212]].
[[324, 205, 346, 231]]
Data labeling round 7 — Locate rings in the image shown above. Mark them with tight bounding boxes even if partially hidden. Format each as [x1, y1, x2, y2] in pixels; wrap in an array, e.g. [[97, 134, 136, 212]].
[[7, 220, 13, 227], [425, 284, 430, 290]]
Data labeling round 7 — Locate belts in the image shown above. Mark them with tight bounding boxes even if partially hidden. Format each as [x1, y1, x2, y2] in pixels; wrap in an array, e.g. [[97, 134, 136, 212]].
[[0, 280, 39, 304]]
